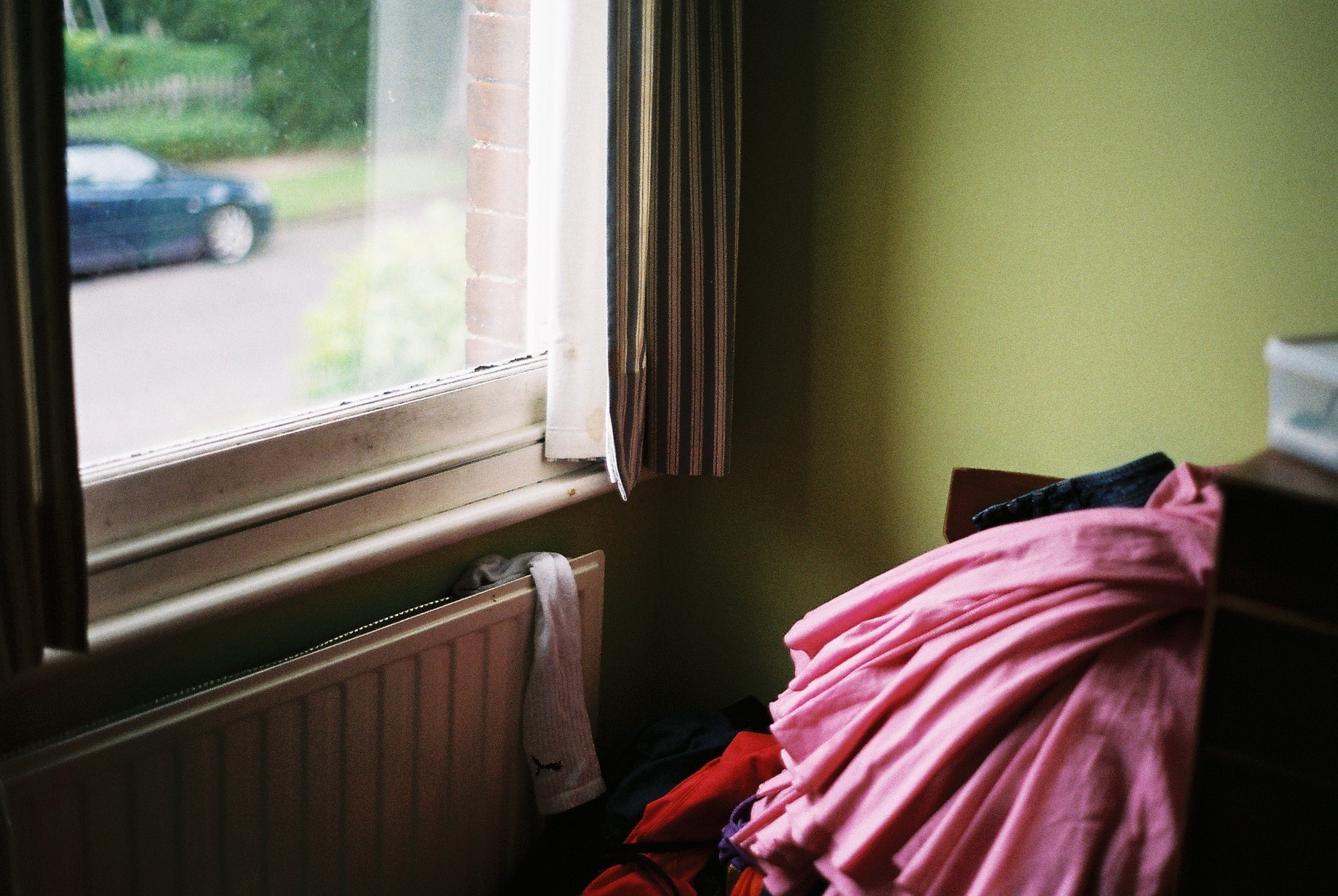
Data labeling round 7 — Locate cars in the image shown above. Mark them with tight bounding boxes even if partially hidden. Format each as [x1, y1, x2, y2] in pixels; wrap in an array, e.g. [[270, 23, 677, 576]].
[[65, 142, 275, 278]]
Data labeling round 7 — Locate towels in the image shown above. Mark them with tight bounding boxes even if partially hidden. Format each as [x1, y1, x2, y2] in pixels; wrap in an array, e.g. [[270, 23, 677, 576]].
[[464, 549, 608, 815]]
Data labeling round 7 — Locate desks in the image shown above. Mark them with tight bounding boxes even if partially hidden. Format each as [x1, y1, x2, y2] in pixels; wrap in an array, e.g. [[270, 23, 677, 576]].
[[1167, 449, 1338, 896]]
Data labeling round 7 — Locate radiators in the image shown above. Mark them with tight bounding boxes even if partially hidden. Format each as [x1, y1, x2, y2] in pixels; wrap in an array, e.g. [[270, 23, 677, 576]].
[[0, 548, 607, 896]]
